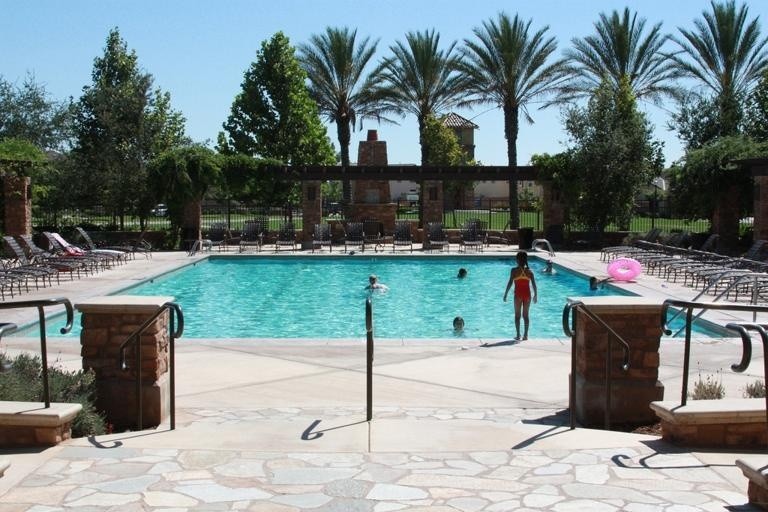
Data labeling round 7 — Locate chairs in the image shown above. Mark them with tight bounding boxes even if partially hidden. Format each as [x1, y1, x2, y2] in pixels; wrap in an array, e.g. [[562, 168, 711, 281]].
[[601, 225, 768, 305], [0, 226, 145, 303]]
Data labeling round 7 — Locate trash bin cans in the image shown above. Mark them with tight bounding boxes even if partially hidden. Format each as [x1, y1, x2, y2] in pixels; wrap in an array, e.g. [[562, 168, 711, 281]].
[[517, 227, 534, 249]]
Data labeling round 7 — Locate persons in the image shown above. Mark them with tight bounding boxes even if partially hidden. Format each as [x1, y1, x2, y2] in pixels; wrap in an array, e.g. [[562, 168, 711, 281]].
[[452, 317, 465, 338], [540, 259, 556, 275], [503, 251, 537, 341], [363, 275, 389, 292], [589, 277, 598, 291], [450, 268, 469, 284]]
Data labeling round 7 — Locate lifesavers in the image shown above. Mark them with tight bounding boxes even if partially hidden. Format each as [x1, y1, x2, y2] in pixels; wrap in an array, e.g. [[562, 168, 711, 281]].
[[609, 258, 641, 282]]
